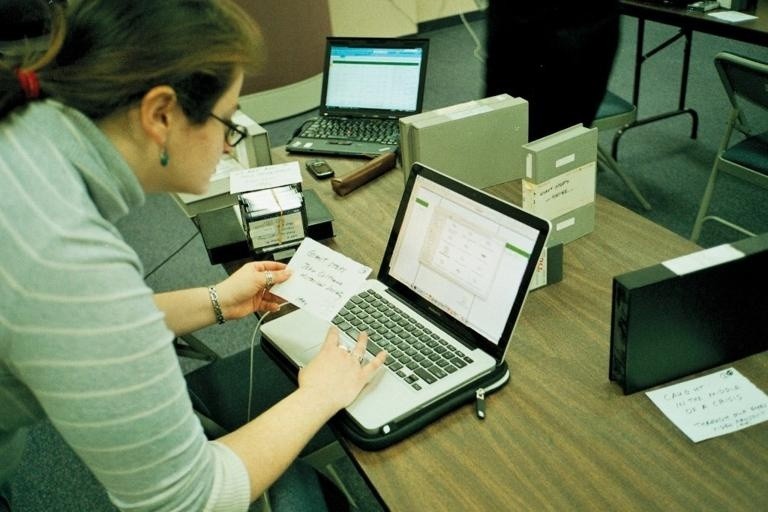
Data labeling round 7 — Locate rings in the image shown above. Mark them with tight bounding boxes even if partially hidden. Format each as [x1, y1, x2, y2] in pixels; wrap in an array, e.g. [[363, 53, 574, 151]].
[[339, 345, 351, 355], [264, 271, 273, 285], [351, 352, 364, 364]]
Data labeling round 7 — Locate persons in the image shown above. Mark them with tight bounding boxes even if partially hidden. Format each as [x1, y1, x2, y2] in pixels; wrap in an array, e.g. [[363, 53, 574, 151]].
[[0, 0, 387, 512]]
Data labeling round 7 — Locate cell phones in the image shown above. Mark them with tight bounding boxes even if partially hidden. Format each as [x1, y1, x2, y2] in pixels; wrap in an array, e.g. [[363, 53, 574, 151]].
[[305, 158, 332, 179]]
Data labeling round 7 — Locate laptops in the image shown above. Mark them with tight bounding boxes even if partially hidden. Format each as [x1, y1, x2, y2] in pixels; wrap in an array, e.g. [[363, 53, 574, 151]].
[[258, 163, 551, 439], [286, 37, 430, 161]]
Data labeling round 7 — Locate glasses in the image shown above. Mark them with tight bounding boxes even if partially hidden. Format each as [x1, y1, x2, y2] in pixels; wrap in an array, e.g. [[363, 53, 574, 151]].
[[203, 109, 248, 147]]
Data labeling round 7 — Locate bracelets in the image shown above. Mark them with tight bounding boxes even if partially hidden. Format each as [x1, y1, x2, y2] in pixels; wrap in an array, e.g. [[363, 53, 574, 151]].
[[209, 287, 226, 325]]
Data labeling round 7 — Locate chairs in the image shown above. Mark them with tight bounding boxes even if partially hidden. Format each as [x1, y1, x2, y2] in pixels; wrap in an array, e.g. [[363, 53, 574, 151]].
[[482, 4, 622, 159], [465, 14, 652, 210], [690, 51, 766, 244]]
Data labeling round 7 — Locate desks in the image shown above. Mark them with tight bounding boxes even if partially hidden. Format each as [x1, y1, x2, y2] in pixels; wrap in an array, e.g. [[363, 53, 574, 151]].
[[222, 143, 768, 509], [610, 2, 765, 165]]
